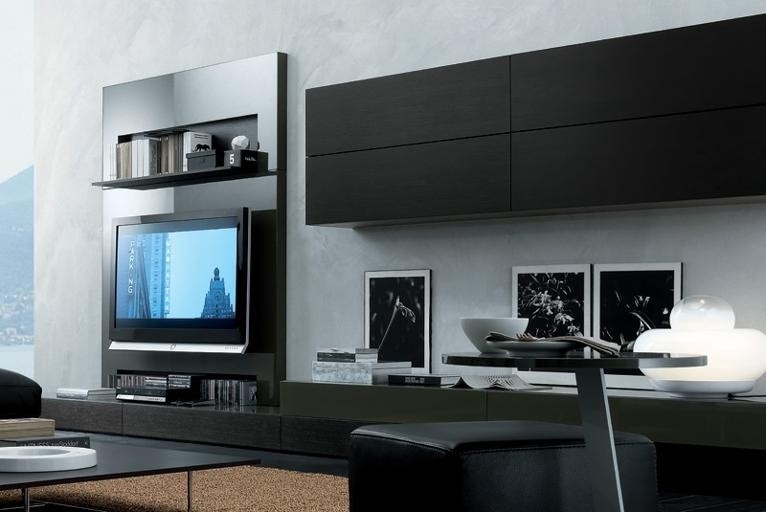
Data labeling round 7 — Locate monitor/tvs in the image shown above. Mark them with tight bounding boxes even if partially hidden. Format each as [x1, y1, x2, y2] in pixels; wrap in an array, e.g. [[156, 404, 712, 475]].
[[107, 206, 254, 355]]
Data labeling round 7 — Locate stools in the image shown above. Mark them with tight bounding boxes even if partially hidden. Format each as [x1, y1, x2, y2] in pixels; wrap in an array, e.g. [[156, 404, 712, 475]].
[[347, 420, 657, 512]]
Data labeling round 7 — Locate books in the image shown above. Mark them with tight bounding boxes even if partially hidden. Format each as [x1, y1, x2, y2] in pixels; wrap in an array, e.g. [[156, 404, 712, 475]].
[[317, 353, 379, 359], [449, 374, 552, 391], [55, 386, 117, 401], [387, 373, 460, 387], [111, 371, 257, 407], [113, 129, 212, 182], [1, 418, 55, 431], [317, 357, 377, 363], [318, 347, 380, 354], [0, 428, 55, 440]]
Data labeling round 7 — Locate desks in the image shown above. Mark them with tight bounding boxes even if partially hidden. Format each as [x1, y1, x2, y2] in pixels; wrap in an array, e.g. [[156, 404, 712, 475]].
[[440, 349, 717, 511], [0, 440, 261, 511]]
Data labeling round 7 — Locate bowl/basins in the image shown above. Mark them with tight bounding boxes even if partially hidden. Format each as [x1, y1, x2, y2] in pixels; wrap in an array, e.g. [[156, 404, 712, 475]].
[[458, 317, 530, 355]]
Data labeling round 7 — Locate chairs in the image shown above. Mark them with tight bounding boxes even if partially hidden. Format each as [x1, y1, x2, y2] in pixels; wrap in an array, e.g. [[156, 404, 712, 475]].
[[0, 368, 42, 418]]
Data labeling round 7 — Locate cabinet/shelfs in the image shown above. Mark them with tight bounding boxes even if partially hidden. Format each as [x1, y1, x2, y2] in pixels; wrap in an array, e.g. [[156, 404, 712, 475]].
[[511, 107, 766, 222], [509, 14, 766, 131], [39, 373, 277, 467], [306, 54, 509, 153], [305, 135, 509, 229], [101, 52, 285, 192], [283, 381, 766, 510]]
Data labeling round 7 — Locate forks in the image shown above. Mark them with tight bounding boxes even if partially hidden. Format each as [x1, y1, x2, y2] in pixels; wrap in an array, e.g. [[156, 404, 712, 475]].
[[514, 331, 546, 342]]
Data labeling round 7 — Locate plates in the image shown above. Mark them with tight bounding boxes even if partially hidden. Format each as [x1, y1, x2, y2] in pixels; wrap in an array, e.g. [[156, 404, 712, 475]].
[[647, 378, 755, 400], [486, 340, 584, 358]]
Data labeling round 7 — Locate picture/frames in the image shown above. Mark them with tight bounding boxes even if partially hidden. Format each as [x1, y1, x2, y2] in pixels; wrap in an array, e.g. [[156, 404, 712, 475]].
[[363, 267, 435, 377], [510, 263, 593, 386], [592, 262, 684, 387]]
